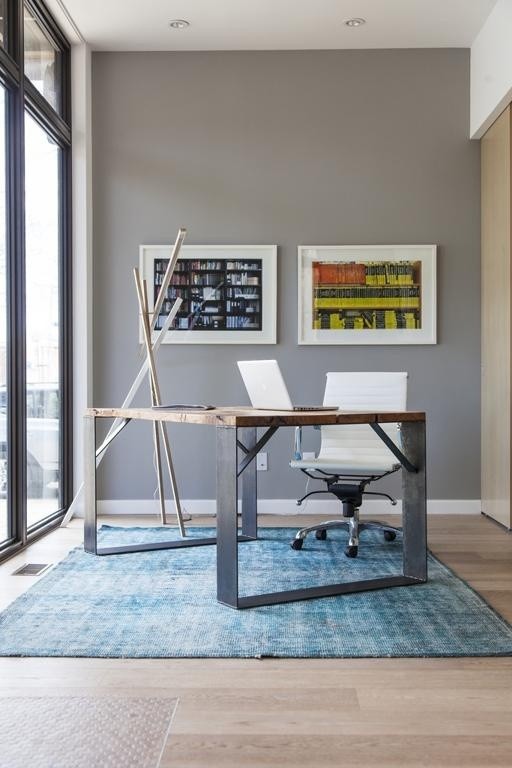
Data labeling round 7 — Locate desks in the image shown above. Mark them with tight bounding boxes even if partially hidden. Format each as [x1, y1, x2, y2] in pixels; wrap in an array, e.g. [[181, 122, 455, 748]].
[[86, 407, 427, 610]]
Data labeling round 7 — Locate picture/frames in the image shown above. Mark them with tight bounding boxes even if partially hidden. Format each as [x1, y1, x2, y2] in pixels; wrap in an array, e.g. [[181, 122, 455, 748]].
[[138, 244, 278, 344], [297, 244, 437, 345]]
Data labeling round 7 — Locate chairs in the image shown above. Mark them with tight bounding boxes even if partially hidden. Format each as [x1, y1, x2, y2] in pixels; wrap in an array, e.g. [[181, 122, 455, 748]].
[[285, 372, 409, 557]]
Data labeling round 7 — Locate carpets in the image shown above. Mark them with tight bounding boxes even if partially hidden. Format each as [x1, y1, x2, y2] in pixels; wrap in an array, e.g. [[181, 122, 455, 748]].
[[1, 524, 512, 658]]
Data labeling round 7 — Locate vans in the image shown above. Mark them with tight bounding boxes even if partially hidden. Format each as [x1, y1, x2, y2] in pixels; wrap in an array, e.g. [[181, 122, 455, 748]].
[[0, 380, 61, 499]]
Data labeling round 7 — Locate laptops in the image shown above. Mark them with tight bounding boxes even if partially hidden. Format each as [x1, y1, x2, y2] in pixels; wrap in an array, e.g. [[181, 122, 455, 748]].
[[236, 359, 341, 412]]
